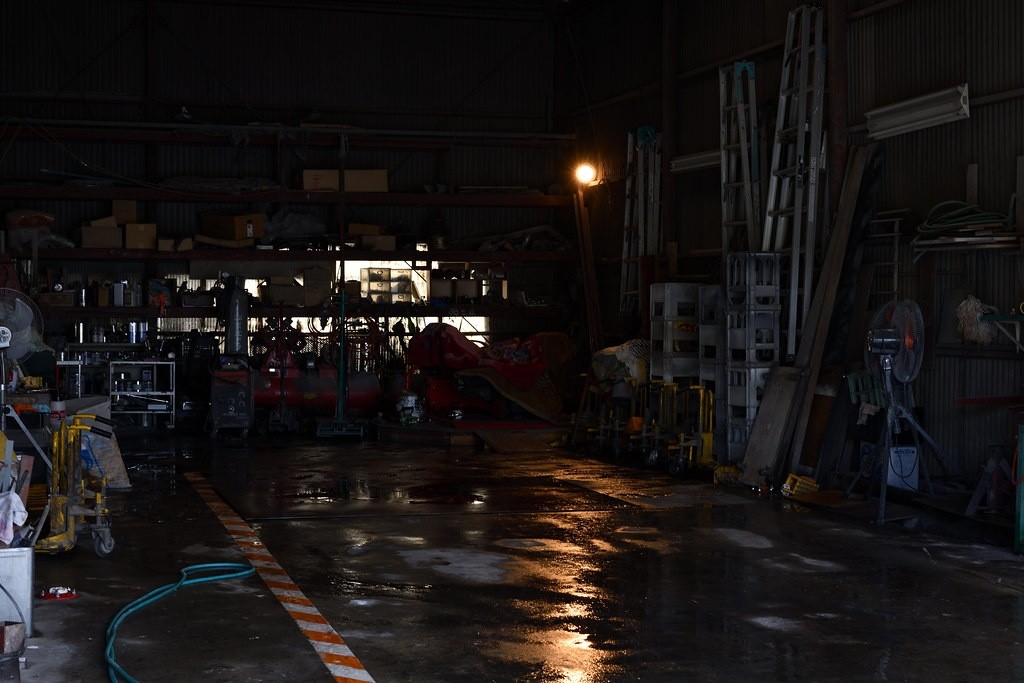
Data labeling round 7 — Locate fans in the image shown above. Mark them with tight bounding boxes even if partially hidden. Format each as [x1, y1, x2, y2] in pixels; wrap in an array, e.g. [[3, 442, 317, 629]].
[[864, 299, 958, 527], [0, 287, 45, 430]]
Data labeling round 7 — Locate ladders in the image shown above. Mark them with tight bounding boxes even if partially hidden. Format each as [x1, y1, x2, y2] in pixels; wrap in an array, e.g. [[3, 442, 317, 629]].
[[758, 3, 824, 363], [620, 124, 662, 309], [718, 58, 764, 287]]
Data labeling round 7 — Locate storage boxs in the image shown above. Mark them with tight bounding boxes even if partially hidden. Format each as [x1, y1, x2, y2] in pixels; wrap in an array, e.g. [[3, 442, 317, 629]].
[[259, 270, 331, 307], [81, 200, 268, 252], [0, 621, 26, 655], [784, 472, 820, 496], [345, 280, 361, 304], [456, 279, 478, 299], [847, 406, 926, 493], [345, 170, 388, 192], [430, 279, 453, 298], [348, 223, 396, 251], [648, 252, 781, 465], [48, 292, 74, 306], [368, 268, 411, 304], [303, 170, 340, 192], [0, 547, 33, 638]]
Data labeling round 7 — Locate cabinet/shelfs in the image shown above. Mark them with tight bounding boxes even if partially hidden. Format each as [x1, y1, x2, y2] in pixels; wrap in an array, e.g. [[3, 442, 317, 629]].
[[110, 361, 175, 429], [2, 121, 601, 437]]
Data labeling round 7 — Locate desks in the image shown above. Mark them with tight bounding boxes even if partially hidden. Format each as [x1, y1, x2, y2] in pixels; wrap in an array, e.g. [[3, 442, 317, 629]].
[[64, 342, 143, 400]]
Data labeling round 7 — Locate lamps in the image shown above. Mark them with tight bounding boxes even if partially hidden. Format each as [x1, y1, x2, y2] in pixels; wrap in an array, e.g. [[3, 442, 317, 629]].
[[864, 83, 969, 142]]
